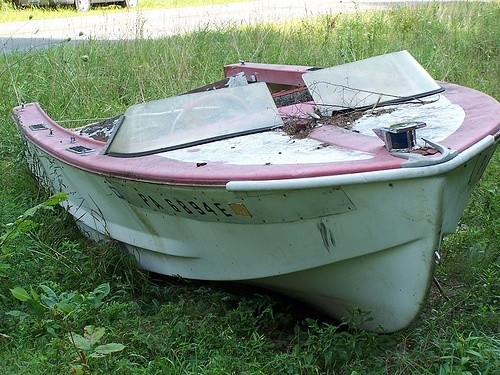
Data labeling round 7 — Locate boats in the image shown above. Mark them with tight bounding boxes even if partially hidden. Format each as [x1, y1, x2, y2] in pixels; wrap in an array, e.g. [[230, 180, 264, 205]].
[[9, 50, 500, 338]]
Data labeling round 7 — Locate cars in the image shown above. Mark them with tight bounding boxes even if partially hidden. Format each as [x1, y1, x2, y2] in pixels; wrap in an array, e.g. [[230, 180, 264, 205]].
[[5, 0, 139, 12]]
